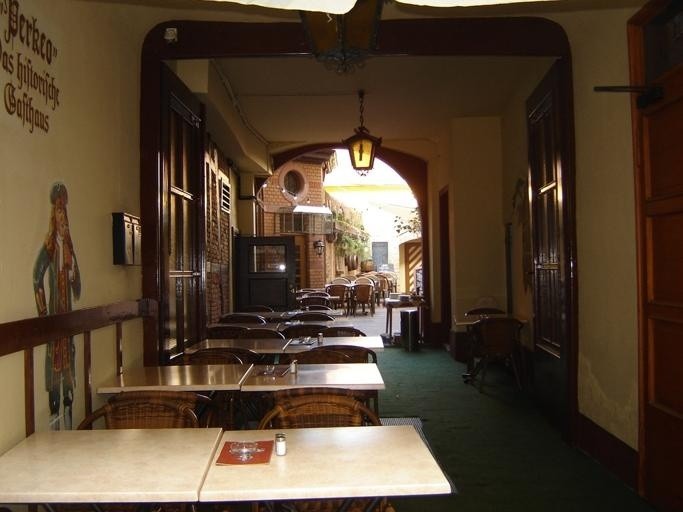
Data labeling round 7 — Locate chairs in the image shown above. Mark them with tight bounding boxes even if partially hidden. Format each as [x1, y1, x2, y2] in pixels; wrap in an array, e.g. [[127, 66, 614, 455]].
[[462, 307, 525, 397]]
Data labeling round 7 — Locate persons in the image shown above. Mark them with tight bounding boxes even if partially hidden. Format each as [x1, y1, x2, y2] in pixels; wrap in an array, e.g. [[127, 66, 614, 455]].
[[31, 181, 82, 429]]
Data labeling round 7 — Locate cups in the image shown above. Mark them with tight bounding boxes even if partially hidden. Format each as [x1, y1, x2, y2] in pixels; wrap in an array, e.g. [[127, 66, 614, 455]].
[[230, 440, 260, 460], [263, 364, 276, 375]]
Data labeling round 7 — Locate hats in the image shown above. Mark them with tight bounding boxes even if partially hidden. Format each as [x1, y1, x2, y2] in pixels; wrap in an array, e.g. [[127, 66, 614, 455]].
[[50, 183, 68, 206]]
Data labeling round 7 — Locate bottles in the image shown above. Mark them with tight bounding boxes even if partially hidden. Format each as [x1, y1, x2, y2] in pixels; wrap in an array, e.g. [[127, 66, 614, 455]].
[[273, 432, 287, 456], [317, 333, 323, 343], [289, 360, 297, 373]]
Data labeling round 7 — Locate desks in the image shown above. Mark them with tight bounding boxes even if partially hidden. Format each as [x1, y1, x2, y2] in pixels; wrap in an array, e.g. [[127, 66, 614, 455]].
[[455, 314, 528, 325]]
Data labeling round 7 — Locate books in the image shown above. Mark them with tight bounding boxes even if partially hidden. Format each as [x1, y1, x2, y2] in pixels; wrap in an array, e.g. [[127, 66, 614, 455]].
[[215, 439, 274, 466], [257, 367, 289, 376], [289, 337, 317, 345]]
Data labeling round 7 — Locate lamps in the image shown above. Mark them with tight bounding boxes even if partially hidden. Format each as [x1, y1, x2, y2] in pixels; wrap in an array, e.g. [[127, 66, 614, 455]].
[[342, 89, 382, 177]]
